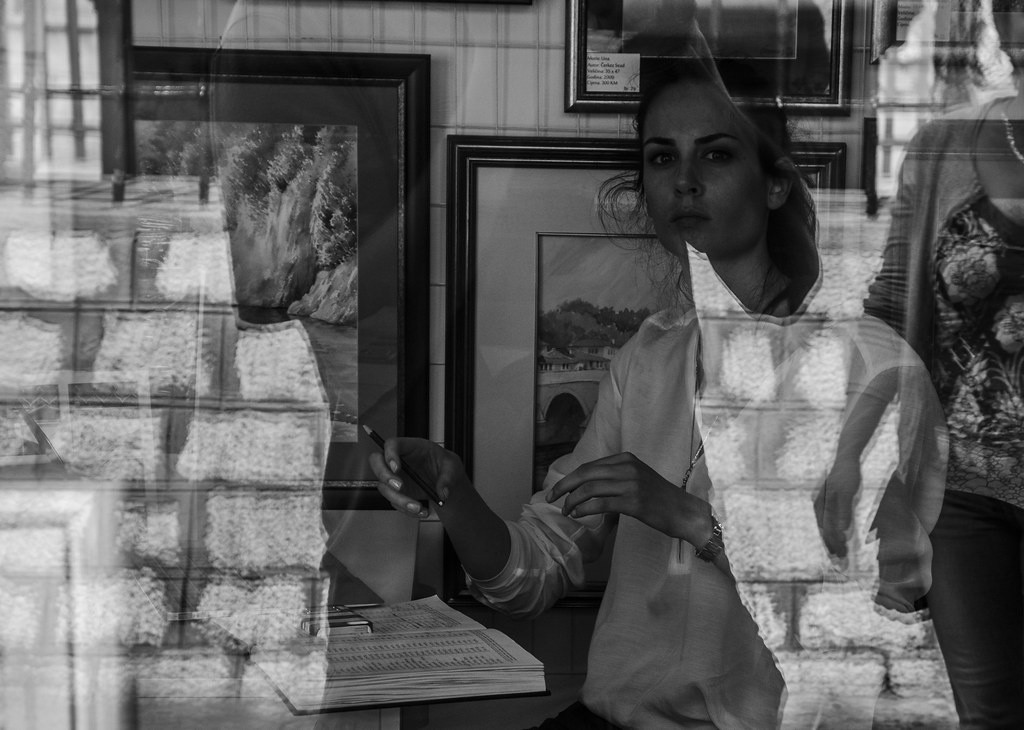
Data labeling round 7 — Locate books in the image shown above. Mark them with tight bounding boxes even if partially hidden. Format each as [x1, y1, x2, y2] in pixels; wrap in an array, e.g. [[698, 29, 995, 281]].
[[197, 592, 551, 722]]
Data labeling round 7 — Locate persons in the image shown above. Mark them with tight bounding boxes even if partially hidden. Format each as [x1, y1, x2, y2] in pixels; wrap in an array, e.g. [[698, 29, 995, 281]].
[[811, 0, 1024, 730], [362, 53, 949, 730]]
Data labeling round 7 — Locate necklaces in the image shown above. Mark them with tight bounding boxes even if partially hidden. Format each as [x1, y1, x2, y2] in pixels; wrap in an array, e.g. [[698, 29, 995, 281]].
[[996, 96, 1024, 166], [666, 255, 818, 561]]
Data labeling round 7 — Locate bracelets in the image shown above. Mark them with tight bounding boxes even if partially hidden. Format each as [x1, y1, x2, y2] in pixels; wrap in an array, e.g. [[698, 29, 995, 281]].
[[694, 497, 734, 568]]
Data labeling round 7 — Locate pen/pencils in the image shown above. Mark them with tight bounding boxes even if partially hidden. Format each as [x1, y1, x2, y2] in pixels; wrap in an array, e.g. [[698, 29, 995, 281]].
[[360, 423, 444, 507]]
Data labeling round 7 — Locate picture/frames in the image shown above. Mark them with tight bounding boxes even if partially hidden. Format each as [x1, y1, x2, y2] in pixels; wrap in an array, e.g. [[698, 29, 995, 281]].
[[562, 0, 858, 118], [438, 132, 849, 609], [120, 45, 433, 514]]
